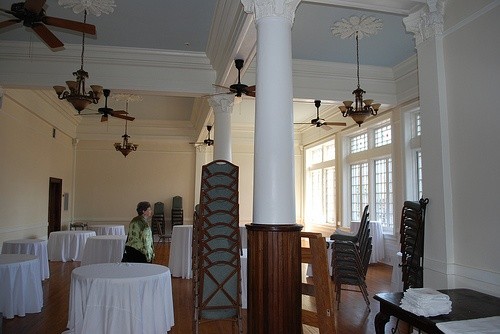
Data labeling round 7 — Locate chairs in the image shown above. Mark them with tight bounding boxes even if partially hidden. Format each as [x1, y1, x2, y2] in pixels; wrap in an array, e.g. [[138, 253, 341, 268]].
[[171, 196, 184, 228], [150, 202, 170, 246], [192, 158, 244, 334], [391, 198, 431, 334], [330, 203, 373, 312]]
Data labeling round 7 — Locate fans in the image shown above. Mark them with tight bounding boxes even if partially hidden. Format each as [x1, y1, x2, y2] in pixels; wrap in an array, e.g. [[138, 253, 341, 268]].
[[0, 0, 96, 48], [204, 58, 256, 98], [293, 100, 346, 132], [188, 126, 215, 147], [76, 89, 134, 123]]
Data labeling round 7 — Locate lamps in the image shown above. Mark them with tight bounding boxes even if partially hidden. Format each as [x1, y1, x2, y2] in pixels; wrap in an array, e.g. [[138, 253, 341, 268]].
[[330, 16, 382, 127], [113, 92, 139, 156], [53, 0, 116, 114]]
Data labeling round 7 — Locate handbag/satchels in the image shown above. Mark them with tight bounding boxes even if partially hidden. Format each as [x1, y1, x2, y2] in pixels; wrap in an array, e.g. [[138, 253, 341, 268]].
[[121, 245, 148, 263]]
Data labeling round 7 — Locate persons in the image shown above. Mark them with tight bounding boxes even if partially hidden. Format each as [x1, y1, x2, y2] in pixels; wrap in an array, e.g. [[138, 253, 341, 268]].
[[121, 201, 155, 263]]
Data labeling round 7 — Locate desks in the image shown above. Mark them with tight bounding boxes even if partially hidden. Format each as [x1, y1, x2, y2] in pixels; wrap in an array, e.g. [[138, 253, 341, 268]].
[[68, 262, 176, 334], [168, 225, 194, 282], [373, 288, 500, 334], [0, 225, 128, 319]]
